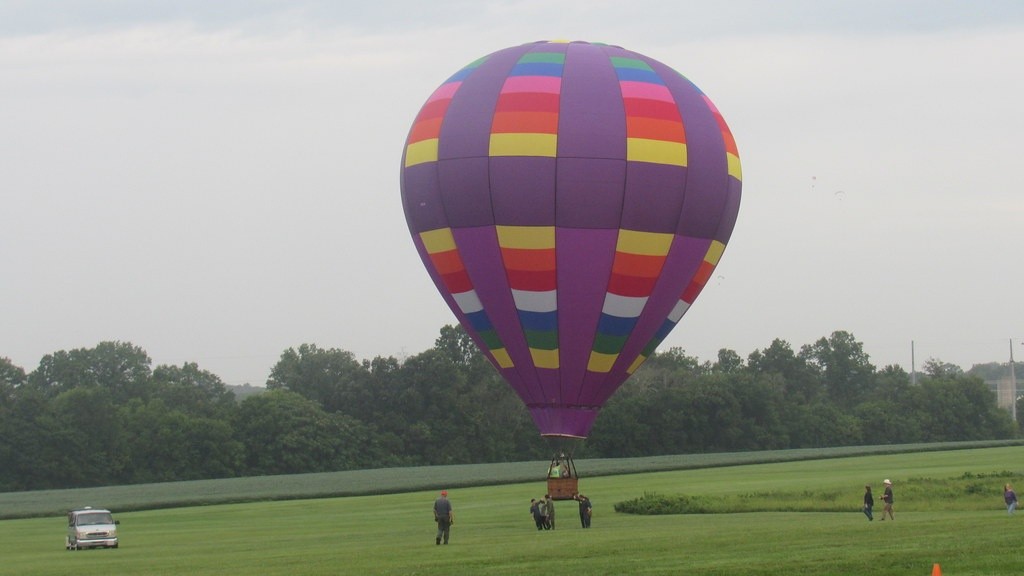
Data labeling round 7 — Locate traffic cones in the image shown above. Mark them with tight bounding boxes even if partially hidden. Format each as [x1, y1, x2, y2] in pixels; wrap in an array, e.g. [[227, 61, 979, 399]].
[[931, 563, 942, 576]]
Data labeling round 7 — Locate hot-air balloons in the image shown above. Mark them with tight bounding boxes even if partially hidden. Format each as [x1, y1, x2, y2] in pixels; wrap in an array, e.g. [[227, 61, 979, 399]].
[[397, 40, 743, 501]]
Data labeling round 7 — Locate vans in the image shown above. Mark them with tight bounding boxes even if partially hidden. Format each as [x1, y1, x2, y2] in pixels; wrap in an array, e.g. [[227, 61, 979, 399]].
[[66, 505, 120, 550]]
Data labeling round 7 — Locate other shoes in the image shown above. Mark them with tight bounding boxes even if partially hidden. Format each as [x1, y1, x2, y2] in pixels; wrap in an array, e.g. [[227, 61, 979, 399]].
[[436, 538, 440, 545], [547, 525, 551, 530]]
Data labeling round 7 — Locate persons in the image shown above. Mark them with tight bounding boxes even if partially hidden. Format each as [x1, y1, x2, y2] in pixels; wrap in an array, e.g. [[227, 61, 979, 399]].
[[548, 462, 570, 478], [862, 486, 874, 521], [878, 479, 895, 521], [1004, 484, 1020, 515], [433, 490, 453, 545], [574, 495, 592, 528], [530, 495, 555, 531]]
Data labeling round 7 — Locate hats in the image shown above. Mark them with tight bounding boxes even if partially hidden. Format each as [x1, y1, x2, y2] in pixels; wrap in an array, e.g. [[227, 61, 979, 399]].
[[884, 480, 891, 484], [441, 491, 448, 495]]
